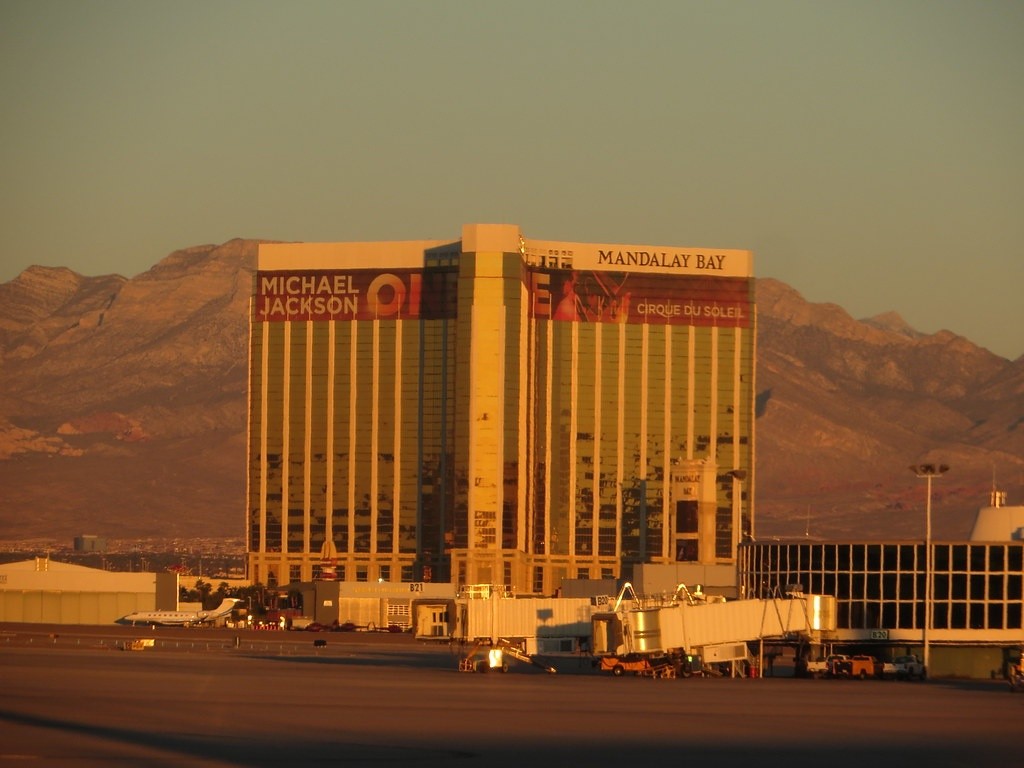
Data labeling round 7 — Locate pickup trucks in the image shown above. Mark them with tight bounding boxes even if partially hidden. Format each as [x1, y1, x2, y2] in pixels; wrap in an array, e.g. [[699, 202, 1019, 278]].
[[882, 654, 927, 681]]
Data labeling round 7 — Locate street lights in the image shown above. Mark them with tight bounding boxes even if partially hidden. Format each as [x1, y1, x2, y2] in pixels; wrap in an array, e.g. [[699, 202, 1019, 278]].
[[909, 461, 953, 671]]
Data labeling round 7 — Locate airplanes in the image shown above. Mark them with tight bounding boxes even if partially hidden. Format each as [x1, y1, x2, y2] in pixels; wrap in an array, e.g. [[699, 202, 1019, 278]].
[[123, 597, 240, 629]]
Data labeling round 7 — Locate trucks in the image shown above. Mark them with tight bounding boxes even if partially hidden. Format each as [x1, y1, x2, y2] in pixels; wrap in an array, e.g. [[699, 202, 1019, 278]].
[[832, 654, 883, 680]]
